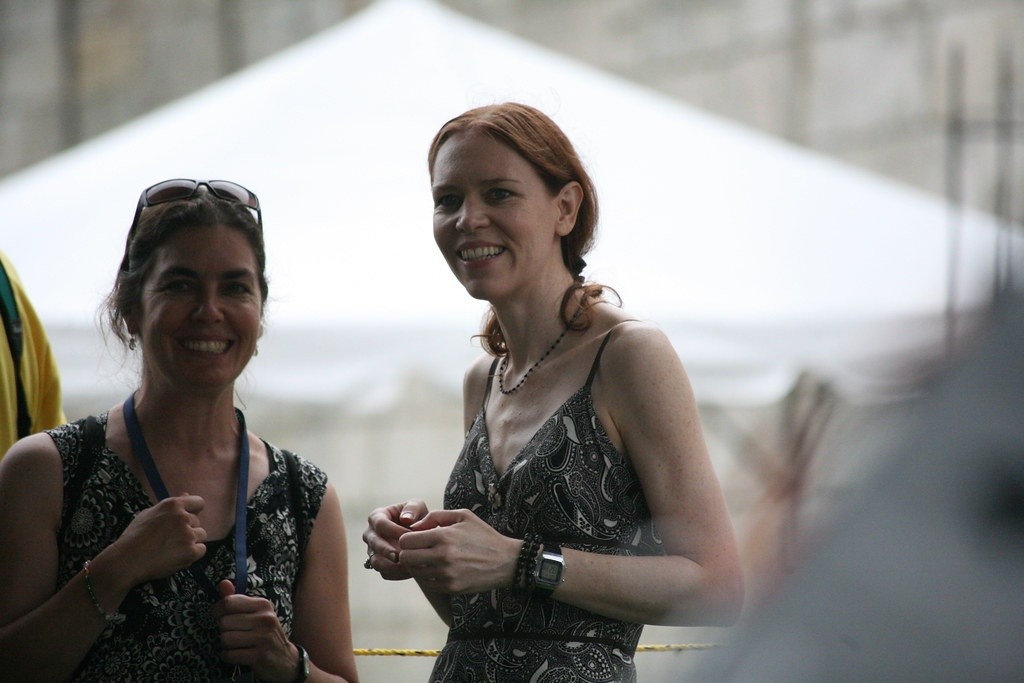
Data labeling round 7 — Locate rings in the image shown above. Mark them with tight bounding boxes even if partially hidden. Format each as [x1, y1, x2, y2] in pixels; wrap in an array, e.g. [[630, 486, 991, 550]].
[[363, 553, 375, 570]]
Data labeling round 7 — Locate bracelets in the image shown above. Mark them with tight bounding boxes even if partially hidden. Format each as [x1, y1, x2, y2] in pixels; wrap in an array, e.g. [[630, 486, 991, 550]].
[[511, 533, 543, 597], [83, 559, 118, 622]]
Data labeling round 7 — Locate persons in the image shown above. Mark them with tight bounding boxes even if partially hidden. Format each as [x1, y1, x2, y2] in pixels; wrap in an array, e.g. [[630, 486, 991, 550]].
[[0, 178, 359, 683], [362, 101, 745, 683]]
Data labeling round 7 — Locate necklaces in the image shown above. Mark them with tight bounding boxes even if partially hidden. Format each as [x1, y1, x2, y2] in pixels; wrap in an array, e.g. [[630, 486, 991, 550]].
[[499, 301, 582, 393]]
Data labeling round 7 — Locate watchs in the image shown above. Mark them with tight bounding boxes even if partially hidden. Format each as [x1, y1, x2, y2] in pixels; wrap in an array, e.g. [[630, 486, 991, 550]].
[[292, 644, 310, 683], [532, 541, 566, 599]]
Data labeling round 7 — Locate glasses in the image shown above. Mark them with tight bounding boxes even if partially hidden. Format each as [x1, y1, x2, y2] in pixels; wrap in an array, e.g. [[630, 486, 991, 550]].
[[132, 178, 262, 238]]
